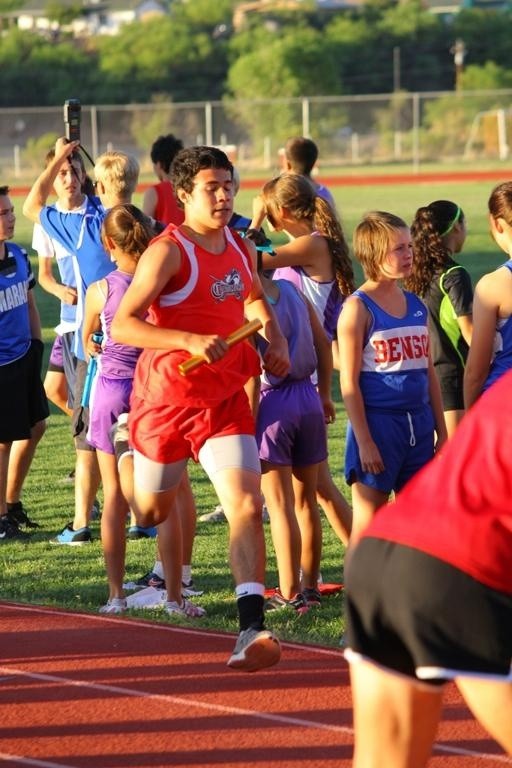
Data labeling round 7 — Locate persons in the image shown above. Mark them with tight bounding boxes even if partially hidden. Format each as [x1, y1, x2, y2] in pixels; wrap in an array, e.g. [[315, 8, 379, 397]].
[[400, 199, 478, 441], [114, 142, 296, 676], [464, 181, 512, 417], [344, 365, 512, 768], [25, 126, 352, 623], [1, 183, 49, 541], [343, 210, 447, 557]]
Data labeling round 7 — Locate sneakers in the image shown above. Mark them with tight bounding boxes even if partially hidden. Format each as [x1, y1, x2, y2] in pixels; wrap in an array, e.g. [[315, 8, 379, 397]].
[[227, 627, 281, 672], [109, 413, 128, 440]]
